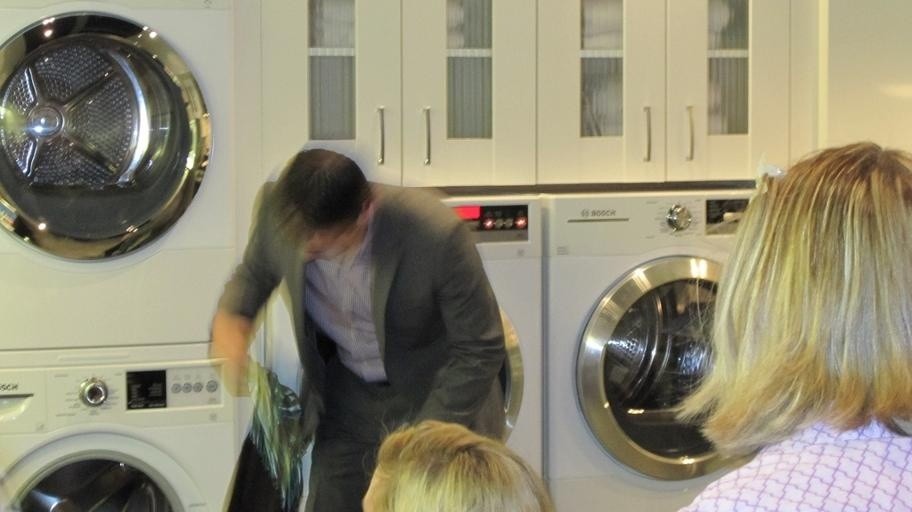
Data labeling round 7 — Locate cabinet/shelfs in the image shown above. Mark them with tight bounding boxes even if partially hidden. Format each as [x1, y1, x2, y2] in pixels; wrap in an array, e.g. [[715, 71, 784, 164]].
[[254, 1, 537, 185], [538, 2, 819, 182]]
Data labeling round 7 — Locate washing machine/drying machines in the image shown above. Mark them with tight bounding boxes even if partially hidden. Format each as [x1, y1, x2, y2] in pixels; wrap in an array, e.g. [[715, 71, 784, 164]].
[[0, 366, 252, 511], [0, 0, 263, 365], [540, 189, 758, 511], [443, 193, 542, 484]]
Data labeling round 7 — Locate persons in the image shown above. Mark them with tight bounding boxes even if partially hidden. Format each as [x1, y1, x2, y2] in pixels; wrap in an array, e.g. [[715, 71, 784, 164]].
[[361, 419, 557, 512], [673, 141, 910, 511], [207, 149, 506, 512]]
